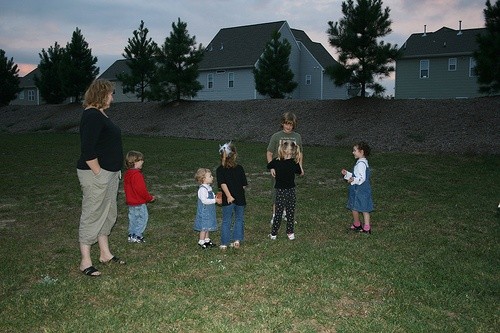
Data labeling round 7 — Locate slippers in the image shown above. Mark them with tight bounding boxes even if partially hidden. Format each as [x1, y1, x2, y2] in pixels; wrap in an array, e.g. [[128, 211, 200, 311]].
[[78, 266, 101, 277], [98, 255, 128, 266]]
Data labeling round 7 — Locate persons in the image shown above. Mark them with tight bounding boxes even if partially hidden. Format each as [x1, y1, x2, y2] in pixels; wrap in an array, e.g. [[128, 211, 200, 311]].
[[216, 142, 249, 249], [193, 168, 219, 249], [124, 151, 155, 243], [265, 142, 304, 240], [76, 79, 126, 275], [342, 141, 375, 234], [267, 112, 303, 224]]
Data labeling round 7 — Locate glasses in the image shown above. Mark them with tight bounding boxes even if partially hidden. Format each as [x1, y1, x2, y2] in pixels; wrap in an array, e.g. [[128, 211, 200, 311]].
[[138, 158, 144, 162]]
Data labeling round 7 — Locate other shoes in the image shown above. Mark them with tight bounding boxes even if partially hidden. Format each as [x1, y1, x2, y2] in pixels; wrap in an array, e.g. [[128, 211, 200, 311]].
[[127, 233, 145, 244], [268, 232, 278, 241], [286, 232, 295, 241], [232, 239, 241, 249], [219, 245, 228, 250], [355, 227, 372, 235], [344, 224, 363, 233], [197, 240, 217, 250]]
